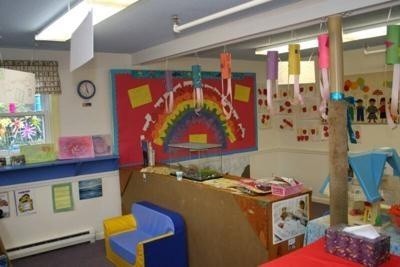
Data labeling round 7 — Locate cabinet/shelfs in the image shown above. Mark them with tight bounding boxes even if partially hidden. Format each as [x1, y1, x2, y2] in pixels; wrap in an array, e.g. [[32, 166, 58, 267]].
[[0, 155, 123, 252], [121, 164, 313, 267]]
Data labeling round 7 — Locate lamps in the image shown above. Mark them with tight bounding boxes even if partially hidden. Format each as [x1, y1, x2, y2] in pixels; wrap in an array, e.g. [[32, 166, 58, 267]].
[[254, 23, 400, 56]]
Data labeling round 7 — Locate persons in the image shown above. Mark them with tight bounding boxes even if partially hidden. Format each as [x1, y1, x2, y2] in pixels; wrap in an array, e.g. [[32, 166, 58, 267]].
[[366, 97, 378, 124], [354, 99, 365, 122], [379, 97, 388, 123]]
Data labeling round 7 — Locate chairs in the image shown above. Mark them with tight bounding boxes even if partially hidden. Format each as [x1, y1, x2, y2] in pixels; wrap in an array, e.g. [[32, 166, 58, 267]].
[[103, 201, 188, 267]]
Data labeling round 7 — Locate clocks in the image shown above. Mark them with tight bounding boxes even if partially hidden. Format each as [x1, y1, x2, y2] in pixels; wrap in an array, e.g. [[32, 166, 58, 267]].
[[77, 80, 96, 100]]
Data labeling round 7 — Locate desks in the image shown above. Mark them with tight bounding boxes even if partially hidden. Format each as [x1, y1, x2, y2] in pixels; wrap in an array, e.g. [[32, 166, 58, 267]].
[[258, 213, 400, 267]]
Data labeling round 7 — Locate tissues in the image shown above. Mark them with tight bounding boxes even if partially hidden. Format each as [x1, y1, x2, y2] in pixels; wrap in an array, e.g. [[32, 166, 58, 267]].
[[325, 222, 391, 267]]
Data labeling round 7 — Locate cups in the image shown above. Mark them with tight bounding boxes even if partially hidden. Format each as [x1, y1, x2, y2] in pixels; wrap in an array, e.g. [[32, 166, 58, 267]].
[[176, 171, 183, 181]]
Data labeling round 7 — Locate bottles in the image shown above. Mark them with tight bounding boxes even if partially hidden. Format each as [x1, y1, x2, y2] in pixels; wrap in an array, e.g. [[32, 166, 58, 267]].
[[35, 93, 43, 112]]
